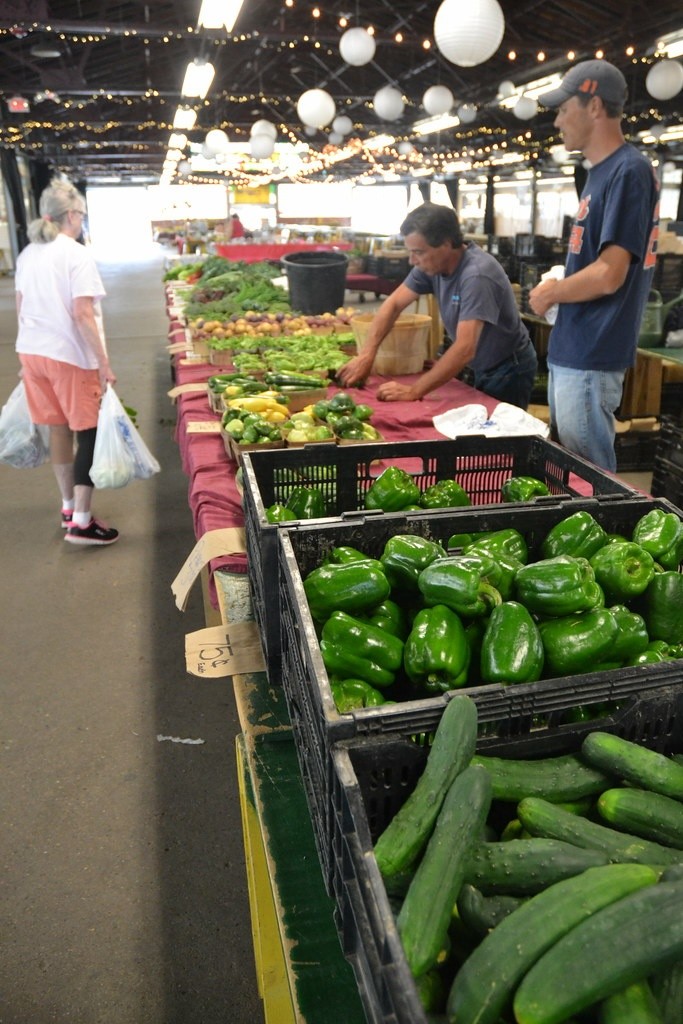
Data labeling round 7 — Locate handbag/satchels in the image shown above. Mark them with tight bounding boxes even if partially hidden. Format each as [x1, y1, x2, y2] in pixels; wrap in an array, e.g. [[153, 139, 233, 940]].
[[106, 381, 162, 481], [89, 382, 135, 490], [0, 380, 51, 469]]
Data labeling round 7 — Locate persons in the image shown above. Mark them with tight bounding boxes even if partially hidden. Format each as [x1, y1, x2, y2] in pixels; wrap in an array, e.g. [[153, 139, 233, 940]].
[[182, 218, 208, 236], [14, 181, 119, 546], [335, 202, 538, 411], [528, 61, 659, 475], [228, 213, 246, 244]]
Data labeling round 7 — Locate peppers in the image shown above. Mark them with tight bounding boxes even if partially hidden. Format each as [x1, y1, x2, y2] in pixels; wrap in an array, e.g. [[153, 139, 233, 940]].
[[265, 464, 552, 523], [303, 509, 683, 713], [204, 333, 357, 371]]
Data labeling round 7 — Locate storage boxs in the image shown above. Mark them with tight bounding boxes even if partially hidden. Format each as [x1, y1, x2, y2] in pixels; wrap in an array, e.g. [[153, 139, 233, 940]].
[[238, 414, 683, 1024], [362, 214, 574, 315], [654, 253, 683, 333]]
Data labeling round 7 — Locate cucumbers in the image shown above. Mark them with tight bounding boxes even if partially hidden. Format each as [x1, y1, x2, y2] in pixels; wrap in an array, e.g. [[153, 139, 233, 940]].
[[372, 693, 683, 1024]]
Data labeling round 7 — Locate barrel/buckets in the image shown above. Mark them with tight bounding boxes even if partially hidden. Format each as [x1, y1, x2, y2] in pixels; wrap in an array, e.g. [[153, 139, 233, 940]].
[[280, 251, 349, 316], [354, 312, 431, 374]]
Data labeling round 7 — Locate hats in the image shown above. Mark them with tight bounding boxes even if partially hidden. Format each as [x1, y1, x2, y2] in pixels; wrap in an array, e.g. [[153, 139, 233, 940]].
[[538, 59, 628, 108]]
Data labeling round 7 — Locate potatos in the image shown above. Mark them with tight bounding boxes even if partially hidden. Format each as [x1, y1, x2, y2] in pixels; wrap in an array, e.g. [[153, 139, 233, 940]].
[[185, 305, 360, 338]]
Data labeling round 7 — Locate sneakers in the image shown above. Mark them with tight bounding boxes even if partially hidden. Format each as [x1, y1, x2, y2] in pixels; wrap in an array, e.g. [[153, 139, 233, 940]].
[[60, 507, 74, 528], [64, 515, 119, 545]]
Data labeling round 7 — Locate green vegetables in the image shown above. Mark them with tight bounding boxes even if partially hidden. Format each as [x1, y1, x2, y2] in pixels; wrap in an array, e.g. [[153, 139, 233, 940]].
[[163, 255, 293, 320]]
